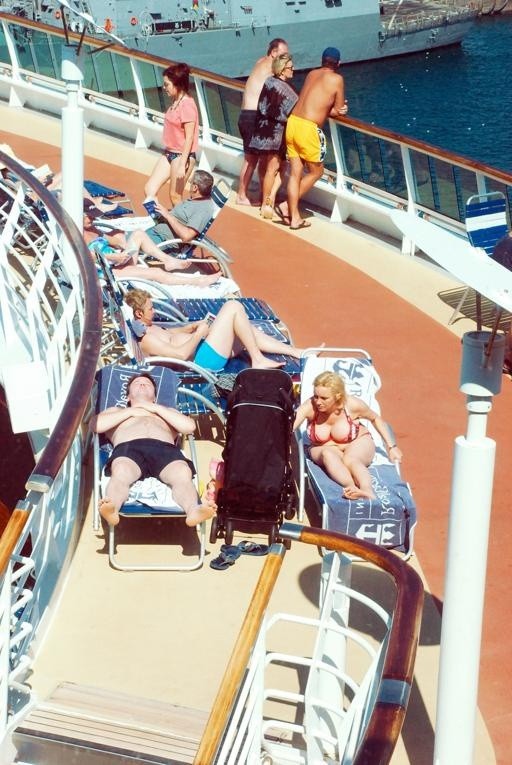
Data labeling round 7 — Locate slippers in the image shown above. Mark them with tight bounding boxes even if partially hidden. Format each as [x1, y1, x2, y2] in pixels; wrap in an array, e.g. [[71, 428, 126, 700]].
[[210, 541, 269, 570], [274, 204, 311, 229]]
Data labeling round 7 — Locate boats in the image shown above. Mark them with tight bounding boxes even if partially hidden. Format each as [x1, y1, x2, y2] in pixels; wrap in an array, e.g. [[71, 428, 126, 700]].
[[0, 0, 473, 92]]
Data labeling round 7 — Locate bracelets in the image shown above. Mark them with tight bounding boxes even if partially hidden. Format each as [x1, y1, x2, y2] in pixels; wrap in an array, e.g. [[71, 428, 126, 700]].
[[388, 444, 398, 451]]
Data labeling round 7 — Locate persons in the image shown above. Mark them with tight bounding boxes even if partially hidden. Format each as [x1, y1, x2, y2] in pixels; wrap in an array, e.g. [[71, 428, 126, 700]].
[[274, 46, 348, 229], [82, 197, 224, 288], [124, 289, 326, 373], [235, 38, 290, 206], [97, 169, 213, 254], [144, 63, 199, 211], [89, 372, 219, 528], [491, 230, 512, 375], [290, 370, 403, 499], [247, 53, 302, 219]]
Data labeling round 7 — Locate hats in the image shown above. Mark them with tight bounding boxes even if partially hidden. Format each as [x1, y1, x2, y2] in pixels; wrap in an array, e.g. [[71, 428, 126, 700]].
[[323, 47, 340, 59]]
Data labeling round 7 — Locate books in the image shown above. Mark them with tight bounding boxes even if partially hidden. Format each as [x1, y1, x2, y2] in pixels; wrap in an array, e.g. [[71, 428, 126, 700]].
[[114, 242, 138, 267], [142, 195, 163, 221], [200, 312, 216, 340]]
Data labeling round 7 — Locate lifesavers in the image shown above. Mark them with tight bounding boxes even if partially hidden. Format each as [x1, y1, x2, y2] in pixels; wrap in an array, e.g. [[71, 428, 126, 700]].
[[130, 17, 137, 25], [54, 11, 62, 19]]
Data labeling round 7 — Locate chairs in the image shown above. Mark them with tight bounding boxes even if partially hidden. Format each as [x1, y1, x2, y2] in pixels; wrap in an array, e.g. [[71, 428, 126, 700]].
[[291, 347, 417, 566], [0, 171, 233, 277], [100, 291, 306, 440], [92, 248, 295, 346], [80, 366, 208, 572]]
